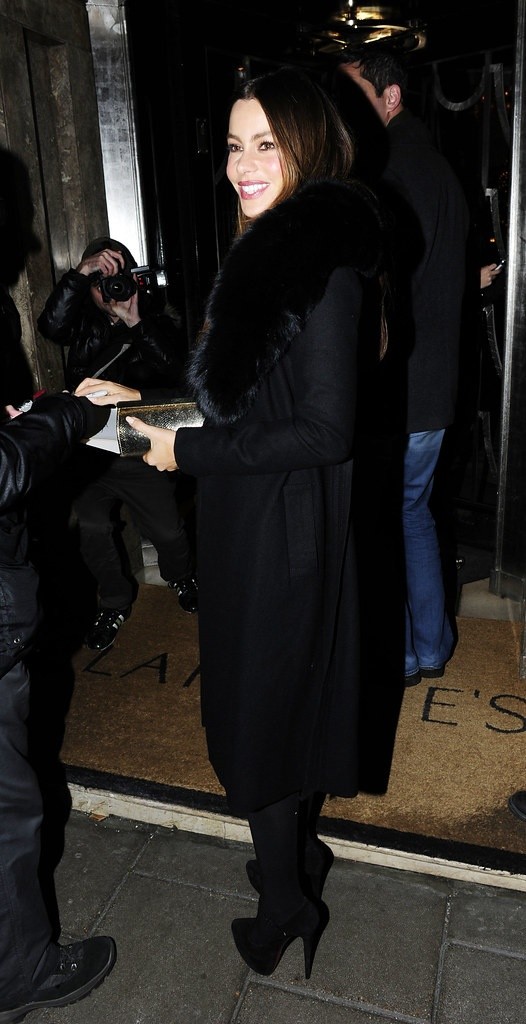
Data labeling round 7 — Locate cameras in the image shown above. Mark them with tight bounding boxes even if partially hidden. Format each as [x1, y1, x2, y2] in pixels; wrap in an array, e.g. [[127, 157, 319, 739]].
[[98, 259, 168, 304]]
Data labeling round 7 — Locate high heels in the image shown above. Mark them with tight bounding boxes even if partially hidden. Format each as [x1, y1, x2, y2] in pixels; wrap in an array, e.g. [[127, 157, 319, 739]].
[[245, 836, 335, 903], [231, 899, 329, 980]]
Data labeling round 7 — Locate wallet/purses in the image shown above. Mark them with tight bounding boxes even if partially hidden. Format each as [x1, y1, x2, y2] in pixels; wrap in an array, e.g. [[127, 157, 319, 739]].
[[117, 400, 205, 457]]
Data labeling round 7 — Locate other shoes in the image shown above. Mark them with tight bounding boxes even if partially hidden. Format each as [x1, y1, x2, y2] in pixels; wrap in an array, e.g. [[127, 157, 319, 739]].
[[509, 791, 526, 821], [419, 665, 444, 678], [405, 670, 421, 688], [0, 935, 116, 1024]]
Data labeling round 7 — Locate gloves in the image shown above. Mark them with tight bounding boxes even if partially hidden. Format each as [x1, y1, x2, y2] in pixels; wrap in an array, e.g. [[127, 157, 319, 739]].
[[77, 396, 115, 440]]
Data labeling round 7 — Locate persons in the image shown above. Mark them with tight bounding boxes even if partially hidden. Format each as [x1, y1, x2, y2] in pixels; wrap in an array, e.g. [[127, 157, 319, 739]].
[[0, 143, 118, 1024], [74, 69, 420, 980], [339, 27, 470, 687], [38, 237, 199, 649], [424, 109, 509, 604]]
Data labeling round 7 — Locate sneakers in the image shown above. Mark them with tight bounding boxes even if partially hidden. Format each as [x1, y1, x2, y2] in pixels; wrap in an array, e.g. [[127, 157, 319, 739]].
[[87, 605, 130, 650], [171, 580, 199, 614]]
